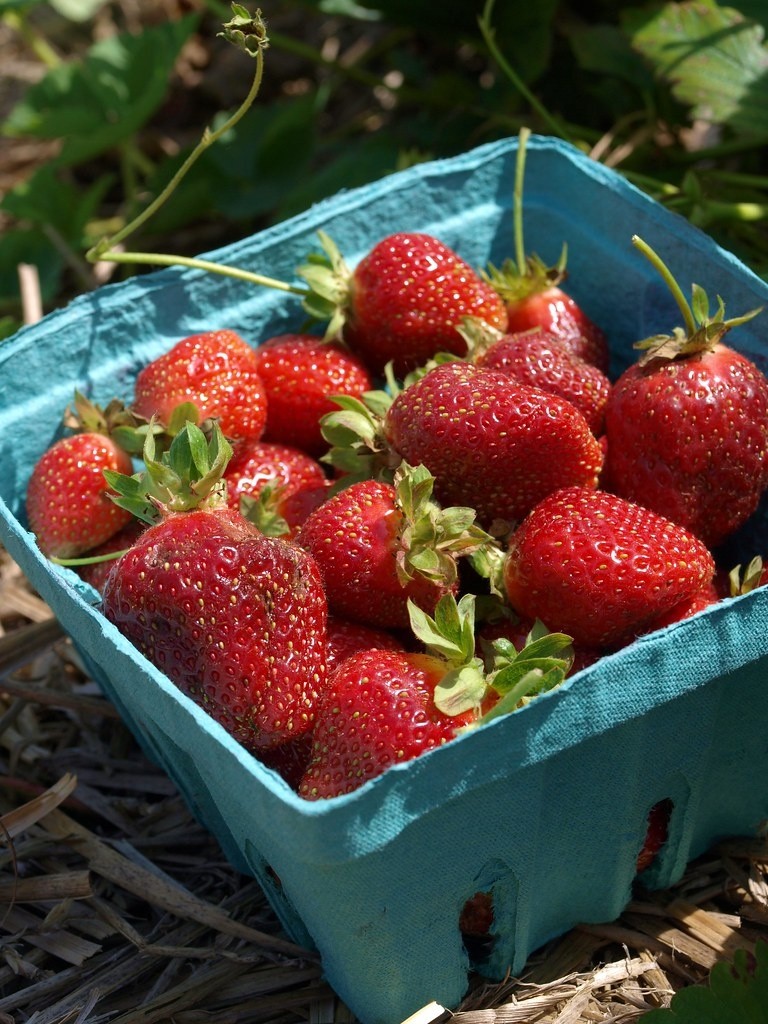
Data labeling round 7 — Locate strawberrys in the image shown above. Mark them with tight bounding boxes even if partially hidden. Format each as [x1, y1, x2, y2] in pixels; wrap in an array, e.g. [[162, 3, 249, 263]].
[[26, 128, 767, 798]]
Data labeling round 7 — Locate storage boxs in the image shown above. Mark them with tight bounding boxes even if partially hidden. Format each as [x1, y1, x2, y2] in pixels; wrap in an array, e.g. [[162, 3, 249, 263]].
[[0, 132, 768, 1024]]
